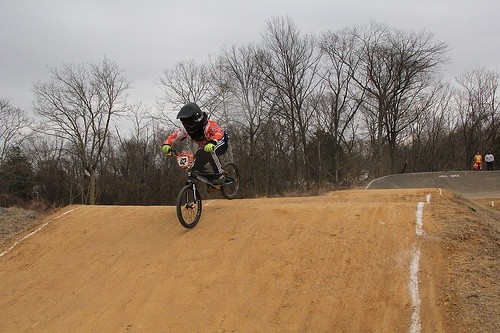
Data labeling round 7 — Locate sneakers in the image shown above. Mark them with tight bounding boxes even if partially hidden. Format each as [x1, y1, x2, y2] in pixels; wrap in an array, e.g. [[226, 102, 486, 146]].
[[212, 174, 226, 185]]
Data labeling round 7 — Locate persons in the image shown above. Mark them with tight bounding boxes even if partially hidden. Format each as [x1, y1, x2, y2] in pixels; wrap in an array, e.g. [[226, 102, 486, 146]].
[[485, 151, 494, 171], [474, 151, 482, 171], [161, 103, 228, 186]]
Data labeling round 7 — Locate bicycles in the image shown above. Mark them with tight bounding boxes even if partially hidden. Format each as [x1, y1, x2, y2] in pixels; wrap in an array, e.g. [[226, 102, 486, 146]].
[[166, 147, 241, 228]]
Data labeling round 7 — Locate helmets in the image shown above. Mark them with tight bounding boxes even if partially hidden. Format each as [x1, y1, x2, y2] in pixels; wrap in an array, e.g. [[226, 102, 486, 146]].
[[175, 102, 202, 127]]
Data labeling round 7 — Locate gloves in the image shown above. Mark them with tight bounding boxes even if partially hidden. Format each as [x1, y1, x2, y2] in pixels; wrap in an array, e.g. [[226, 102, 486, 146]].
[[204, 143, 214, 153], [162, 144, 172, 155]]
